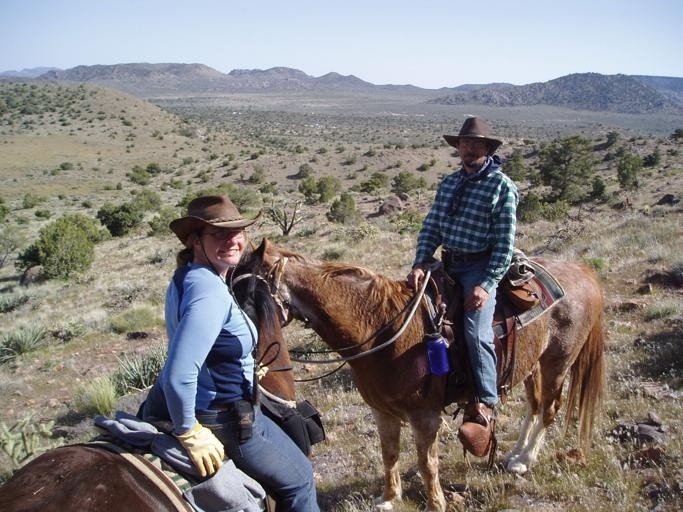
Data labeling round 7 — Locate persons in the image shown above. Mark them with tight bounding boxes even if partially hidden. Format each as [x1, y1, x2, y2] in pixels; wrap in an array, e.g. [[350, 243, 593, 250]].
[[405, 114, 521, 463], [135, 193, 328, 512]]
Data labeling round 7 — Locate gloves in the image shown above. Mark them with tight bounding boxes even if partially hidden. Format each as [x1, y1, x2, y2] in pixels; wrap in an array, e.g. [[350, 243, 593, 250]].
[[172, 418, 226, 479]]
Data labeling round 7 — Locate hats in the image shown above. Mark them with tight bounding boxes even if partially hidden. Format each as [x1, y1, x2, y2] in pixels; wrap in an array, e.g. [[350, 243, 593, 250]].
[[168, 193, 263, 249], [441, 115, 504, 158]]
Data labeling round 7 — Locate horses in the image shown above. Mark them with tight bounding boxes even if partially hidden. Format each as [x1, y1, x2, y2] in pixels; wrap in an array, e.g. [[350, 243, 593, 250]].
[[0, 235, 297, 512], [237, 236, 608, 512]]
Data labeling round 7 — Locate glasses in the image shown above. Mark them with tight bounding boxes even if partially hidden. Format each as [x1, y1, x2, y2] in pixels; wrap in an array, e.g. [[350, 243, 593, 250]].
[[454, 141, 487, 149], [194, 229, 246, 241]]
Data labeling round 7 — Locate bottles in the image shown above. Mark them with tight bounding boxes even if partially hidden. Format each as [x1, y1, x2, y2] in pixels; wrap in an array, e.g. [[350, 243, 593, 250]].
[[424, 332, 450, 375]]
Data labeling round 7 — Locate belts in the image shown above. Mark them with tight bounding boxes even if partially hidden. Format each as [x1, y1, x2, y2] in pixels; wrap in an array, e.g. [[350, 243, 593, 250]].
[[447, 249, 492, 263]]
